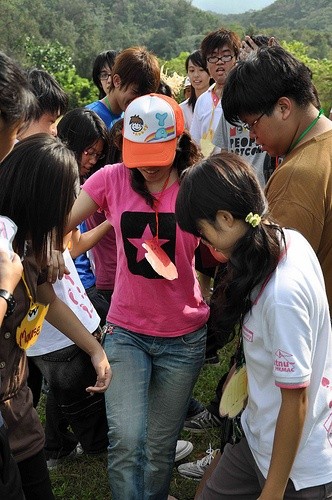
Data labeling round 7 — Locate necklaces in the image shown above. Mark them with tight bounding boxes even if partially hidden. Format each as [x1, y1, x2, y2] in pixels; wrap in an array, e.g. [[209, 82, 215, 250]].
[[104, 96, 111, 112], [278, 111, 323, 164]]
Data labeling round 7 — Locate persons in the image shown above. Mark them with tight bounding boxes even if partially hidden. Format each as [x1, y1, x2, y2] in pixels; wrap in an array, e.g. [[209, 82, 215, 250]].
[[0, 54, 24, 327], [175, 153, 332, 500], [0, 133, 112, 500], [178, 46, 332, 482], [32, 93, 231, 500], [22, 37, 321, 473], [190, 29, 267, 301]]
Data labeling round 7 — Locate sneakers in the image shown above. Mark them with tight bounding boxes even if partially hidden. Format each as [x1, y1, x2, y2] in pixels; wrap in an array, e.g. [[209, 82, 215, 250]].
[[184, 410, 223, 431], [177, 449, 220, 480]]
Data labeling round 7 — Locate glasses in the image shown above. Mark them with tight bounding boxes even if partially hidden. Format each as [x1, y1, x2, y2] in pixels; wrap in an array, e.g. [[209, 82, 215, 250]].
[[206, 55, 234, 63], [240, 112, 264, 131], [84, 151, 106, 159], [100, 72, 109, 80]]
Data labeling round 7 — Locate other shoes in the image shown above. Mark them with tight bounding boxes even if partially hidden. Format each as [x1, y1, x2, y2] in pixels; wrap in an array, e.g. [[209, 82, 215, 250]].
[[174, 440, 193, 460], [86, 448, 108, 461], [202, 287, 213, 305], [185, 398, 205, 419], [48, 445, 84, 468], [203, 356, 220, 369]]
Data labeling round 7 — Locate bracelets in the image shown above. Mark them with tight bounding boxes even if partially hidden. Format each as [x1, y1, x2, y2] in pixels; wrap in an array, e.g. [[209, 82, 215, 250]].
[[0, 289, 17, 316]]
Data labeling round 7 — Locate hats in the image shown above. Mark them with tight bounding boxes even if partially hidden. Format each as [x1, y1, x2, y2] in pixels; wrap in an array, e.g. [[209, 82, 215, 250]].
[[122, 92, 184, 168]]
